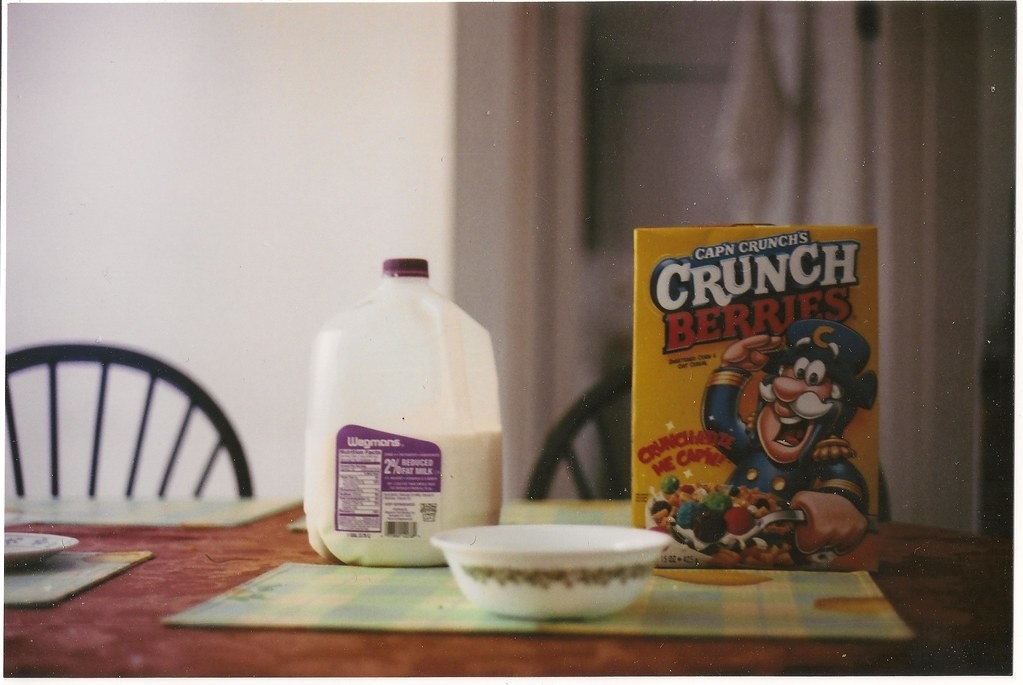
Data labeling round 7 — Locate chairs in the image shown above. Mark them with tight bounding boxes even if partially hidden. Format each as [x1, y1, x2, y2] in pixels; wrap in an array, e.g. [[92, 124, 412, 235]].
[[524, 364, 891, 526], [4, 343, 251, 500]]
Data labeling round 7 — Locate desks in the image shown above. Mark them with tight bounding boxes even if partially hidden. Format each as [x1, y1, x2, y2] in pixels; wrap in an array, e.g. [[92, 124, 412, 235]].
[[4, 499, 1013, 676]]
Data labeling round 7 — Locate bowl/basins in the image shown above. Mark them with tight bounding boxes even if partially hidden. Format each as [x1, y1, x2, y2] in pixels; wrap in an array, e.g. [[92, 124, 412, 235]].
[[427, 523, 672, 621]]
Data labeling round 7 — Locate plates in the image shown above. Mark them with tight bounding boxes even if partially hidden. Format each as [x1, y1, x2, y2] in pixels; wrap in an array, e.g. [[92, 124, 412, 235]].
[[4, 531, 79, 569]]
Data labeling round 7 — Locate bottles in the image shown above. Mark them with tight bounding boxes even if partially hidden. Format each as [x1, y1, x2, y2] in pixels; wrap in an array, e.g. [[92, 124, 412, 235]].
[[303, 257, 504, 565]]
